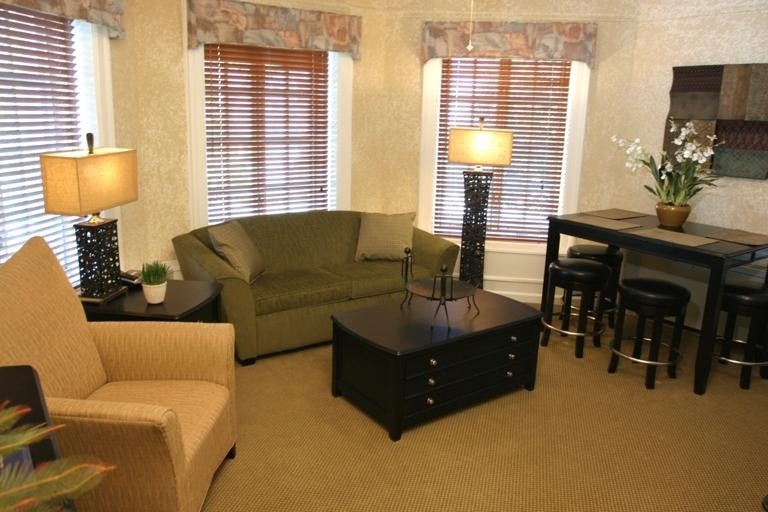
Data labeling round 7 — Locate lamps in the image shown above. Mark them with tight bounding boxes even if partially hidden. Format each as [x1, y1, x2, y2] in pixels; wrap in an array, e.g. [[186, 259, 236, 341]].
[[448, 117, 514, 286], [40, 134, 140, 303]]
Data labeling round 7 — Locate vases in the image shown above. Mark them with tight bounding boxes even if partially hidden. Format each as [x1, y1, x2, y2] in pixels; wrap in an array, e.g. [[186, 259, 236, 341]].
[[655, 204, 693, 229]]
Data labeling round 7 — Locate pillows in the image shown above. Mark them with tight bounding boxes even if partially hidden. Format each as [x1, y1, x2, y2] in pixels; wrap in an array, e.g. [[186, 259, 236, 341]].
[[207, 221, 266, 284], [354, 211, 417, 261]]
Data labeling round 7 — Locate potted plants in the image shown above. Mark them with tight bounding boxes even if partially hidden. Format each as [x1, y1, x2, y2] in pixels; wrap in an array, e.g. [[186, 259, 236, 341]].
[[138, 262, 172, 305]]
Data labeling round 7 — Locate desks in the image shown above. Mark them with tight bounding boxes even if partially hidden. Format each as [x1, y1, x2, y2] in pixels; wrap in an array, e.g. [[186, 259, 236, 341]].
[[85, 278, 224, 322], [539, 207, 767, 388]]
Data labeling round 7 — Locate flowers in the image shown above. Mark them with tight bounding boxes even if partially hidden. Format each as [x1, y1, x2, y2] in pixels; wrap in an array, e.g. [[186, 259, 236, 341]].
[[611, 112, 725, 202]]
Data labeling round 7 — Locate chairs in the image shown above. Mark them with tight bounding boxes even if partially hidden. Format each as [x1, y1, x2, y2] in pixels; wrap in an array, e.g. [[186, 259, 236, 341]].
[[0, 236, 237, 512]]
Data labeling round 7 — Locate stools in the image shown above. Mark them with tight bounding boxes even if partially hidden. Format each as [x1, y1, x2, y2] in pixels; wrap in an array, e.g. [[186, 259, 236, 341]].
[[543, 260, 611, 358], [714, 283, 767, 388], [606, 275, 691, 387], [560, 243, 622, 336]]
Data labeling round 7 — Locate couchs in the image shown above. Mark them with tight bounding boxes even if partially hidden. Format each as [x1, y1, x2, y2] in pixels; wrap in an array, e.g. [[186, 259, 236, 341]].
[[171, 211, 460, 365]]
